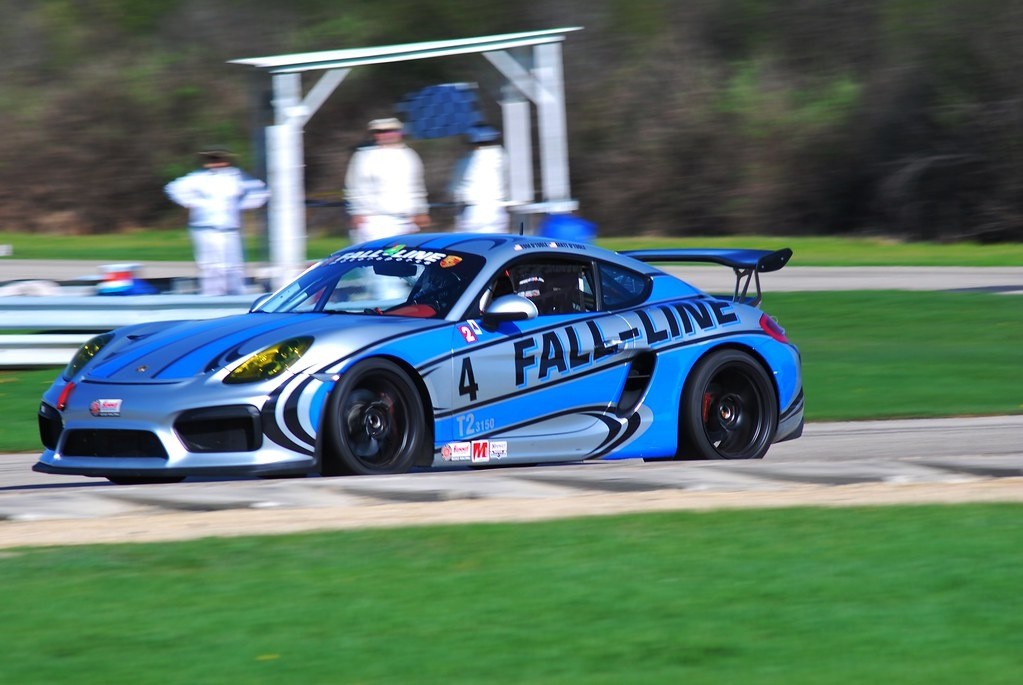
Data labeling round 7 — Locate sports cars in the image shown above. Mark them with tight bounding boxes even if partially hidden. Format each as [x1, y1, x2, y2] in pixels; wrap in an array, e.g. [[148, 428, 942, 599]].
[[31, 233, 804, 483]]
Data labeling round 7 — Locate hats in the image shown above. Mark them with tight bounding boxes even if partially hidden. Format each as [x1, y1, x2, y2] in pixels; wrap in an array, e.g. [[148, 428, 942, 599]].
[[368, 117, 403, 130], [466, 125, 502, 143]]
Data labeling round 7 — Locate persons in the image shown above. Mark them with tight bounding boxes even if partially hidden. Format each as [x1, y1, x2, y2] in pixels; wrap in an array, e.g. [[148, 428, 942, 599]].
[[343, 118, 430, 245], [164, 147, 270, 295], [443, 125, 511, 234]]
